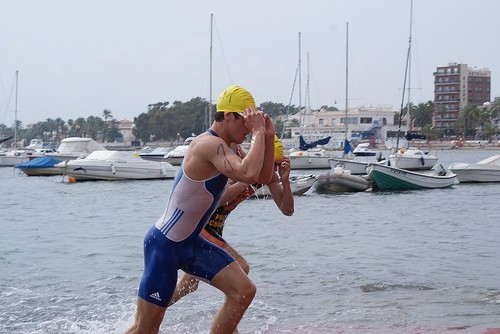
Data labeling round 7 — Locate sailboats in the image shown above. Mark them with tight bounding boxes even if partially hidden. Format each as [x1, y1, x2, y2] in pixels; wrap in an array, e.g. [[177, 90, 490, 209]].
[[390, 0, 438, 170]]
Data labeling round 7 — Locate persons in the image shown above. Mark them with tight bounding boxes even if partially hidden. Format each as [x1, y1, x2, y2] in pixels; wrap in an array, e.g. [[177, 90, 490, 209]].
[[123, 85, 274, 334], [168, 134, 294, 307], [370, 134, 377, 149]]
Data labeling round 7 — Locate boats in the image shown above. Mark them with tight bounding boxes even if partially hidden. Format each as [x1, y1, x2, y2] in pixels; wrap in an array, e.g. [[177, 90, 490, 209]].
[[251, 173, 319, 199], [313, 162, 369, 193], [365, 162, 460, 191], [14, 137, 109, 177], [54, 150, 177, 181], [0, 14, 388, 167], [447, 154, 500, 182]]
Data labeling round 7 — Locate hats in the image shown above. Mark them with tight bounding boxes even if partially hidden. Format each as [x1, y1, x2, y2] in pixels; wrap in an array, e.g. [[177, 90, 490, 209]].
[[216, 85, 257, 112], [274, 135, 284, 163]]
